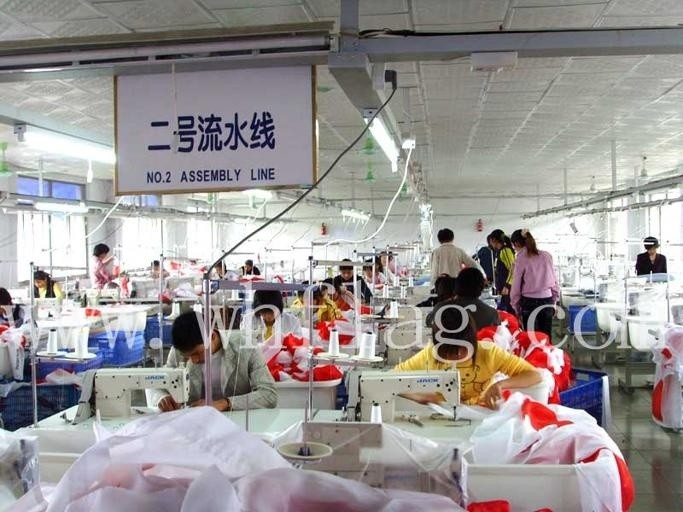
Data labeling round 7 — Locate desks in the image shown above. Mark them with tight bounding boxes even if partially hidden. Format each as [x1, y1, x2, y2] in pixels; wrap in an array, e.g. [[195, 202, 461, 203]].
[[0, 278, 683, 512]]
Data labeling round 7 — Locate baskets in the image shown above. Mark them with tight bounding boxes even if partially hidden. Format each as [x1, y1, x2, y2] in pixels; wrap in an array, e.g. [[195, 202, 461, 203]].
[[567, 304, 598, 333], [88, 330, 145, 366], [0, 383, 81, 433], [146, 317, 175, 345], [559, 365, 607, 410], [34, 347, 104, 379]]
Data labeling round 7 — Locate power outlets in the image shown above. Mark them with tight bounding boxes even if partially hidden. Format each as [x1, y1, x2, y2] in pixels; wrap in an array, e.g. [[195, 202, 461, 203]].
[[371, 63, 386, 90]]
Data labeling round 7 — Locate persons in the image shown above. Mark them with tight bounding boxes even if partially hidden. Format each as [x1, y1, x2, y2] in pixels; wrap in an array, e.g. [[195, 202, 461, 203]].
[[635, 236, 668, 275], [0, 226, 558, 411]]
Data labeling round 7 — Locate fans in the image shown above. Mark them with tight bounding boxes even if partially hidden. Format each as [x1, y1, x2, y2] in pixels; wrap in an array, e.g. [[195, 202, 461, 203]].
[[582, 177, 612, 193], [638, 161, 666, 181]]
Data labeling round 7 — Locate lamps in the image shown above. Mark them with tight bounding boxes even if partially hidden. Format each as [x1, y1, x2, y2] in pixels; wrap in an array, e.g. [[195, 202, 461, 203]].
[[13, 107, 401, 222]]
